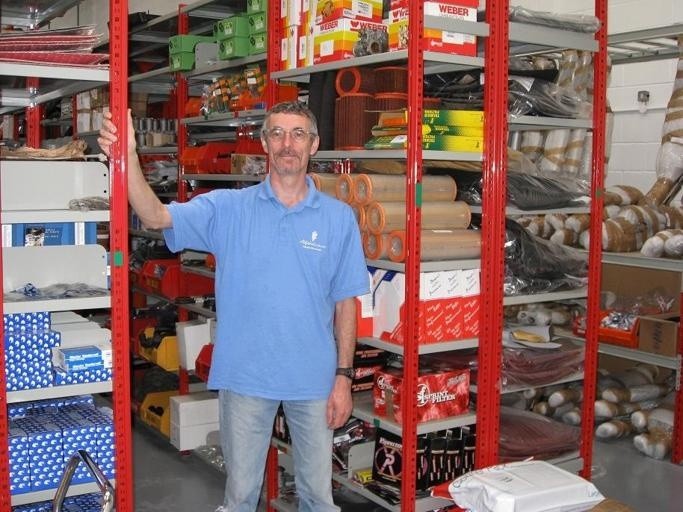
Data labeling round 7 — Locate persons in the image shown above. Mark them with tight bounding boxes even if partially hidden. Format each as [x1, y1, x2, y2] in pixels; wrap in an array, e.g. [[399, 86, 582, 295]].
[[94, 97, 372, 512]]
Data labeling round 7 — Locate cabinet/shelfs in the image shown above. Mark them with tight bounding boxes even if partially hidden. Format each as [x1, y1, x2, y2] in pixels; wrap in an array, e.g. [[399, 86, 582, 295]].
[[0, 1, 135, 511], [491, 0, 683, 511], [129, 0, 499, 512]]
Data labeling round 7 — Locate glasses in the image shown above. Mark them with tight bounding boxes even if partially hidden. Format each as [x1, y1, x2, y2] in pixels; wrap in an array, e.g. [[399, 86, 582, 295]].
[[262, 125, 318, 144]]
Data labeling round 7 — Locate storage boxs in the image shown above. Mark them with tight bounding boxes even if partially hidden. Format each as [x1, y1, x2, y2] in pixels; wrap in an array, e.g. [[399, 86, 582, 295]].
[[364, 109, 490, 158], [326, 264, 489, 512], [2, 2, 118, 510], [167, 0, 478, 72], [636, 310, 681, 359]]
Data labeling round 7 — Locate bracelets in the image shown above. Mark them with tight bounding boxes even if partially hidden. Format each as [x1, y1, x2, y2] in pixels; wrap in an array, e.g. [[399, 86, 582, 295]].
[[335, 366, 355, 379]]
